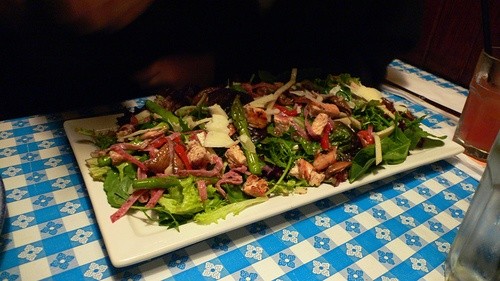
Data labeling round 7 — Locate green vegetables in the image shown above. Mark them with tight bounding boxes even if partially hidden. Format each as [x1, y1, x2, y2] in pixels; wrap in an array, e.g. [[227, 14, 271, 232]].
[[76, 74, 448, 231]]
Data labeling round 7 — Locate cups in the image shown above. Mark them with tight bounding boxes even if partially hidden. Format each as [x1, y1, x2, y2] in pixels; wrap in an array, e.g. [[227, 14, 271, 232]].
[[448, 130, 499, 281], [452, 45, 500, 160]]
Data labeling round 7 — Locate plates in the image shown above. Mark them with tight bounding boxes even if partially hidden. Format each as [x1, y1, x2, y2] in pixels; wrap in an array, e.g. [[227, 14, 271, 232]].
[[63, 112, 466, 268]]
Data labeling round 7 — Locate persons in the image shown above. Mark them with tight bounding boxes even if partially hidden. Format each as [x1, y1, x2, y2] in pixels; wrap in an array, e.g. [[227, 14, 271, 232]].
[[48, 0, 263, 109], [134, 1, 422, 93]]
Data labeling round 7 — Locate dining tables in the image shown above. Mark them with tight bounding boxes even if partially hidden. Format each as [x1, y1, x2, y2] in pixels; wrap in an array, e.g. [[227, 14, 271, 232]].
[[1, 59, 488, 281]]
